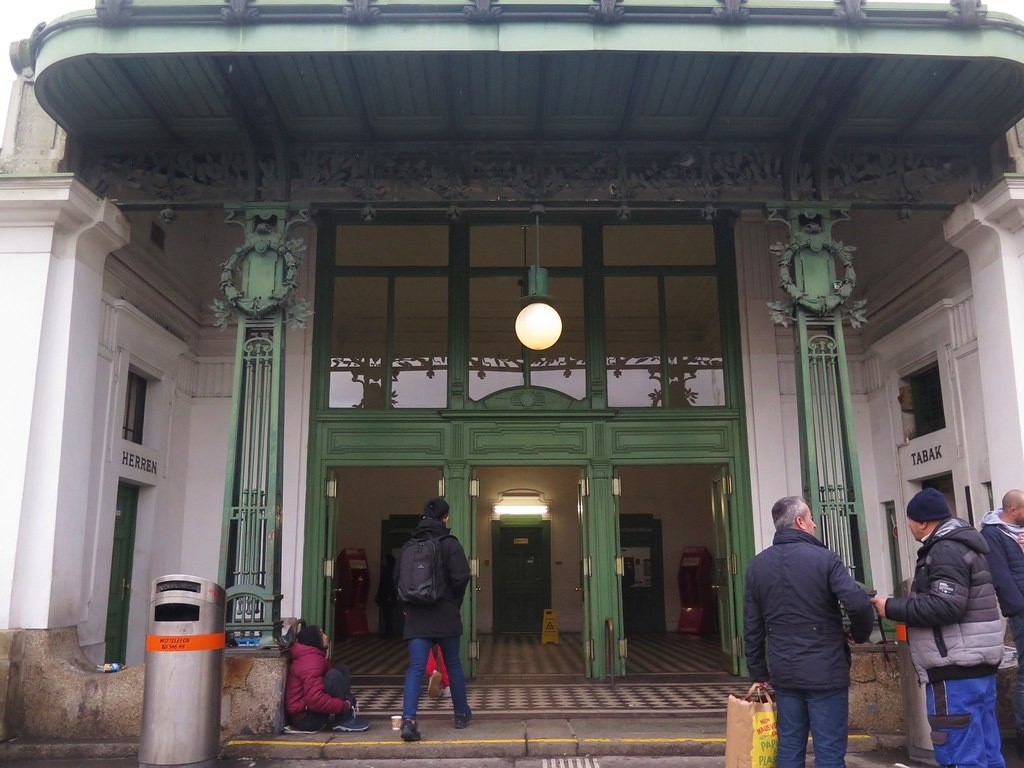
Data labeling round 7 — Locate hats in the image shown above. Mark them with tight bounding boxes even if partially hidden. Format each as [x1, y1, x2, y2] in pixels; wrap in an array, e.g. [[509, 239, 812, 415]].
[[297, 625, 323, 649], [907, 487, 952, 521]]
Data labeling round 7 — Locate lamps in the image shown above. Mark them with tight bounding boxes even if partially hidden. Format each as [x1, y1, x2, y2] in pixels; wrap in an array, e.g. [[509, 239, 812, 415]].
[[515, 204, 562, 350]]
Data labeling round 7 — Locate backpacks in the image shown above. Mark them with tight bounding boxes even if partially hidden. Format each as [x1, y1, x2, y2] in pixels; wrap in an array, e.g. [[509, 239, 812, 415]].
[[396, 534, 459, 607]]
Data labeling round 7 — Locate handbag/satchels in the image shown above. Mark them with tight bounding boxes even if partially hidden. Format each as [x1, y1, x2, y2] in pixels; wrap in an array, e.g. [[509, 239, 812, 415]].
[[725, 683, 777, 768]]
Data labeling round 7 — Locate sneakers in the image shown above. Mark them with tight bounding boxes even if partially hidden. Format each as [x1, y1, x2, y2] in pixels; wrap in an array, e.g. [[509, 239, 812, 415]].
[[331, 719, 369, 732]]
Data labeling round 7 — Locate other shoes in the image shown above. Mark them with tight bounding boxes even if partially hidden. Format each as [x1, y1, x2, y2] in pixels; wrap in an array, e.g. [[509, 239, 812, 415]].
[[443, 687, 451, 697], [400, 720, 421, 741], [454, 708, 472, 729], [428, 672, 443, 697]]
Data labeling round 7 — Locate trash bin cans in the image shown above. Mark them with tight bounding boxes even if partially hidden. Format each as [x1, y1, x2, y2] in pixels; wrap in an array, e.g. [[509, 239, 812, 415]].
[[893, 576, 939, 764], [137, 574, 227, 768]]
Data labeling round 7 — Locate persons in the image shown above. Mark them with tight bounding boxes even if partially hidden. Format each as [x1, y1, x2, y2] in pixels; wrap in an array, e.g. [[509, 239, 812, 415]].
[[374, 553, 399, 639], [392, 497, 472, 741], [743, 496, 875, 768], [285, 625, 369, 732], [870, 487, 1005, 768], [980, 490, 1024, 758], [425, 643, 451, 698]]
